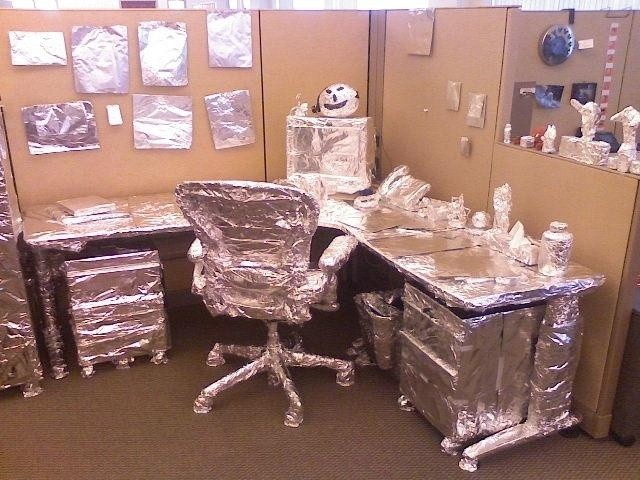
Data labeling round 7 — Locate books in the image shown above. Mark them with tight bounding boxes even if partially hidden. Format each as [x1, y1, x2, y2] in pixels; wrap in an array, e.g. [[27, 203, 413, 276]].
[[46, 207, 129, 225], [55, 195, 117, 217]]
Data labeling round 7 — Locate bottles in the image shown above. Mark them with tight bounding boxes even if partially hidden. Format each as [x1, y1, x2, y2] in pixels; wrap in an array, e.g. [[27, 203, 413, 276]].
[[538, 221, 574, 277]]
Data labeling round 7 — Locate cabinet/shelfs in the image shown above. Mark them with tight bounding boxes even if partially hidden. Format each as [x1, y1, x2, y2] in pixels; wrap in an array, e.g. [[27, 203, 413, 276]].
[[63, 245, 173, 380], [0, 103, 50, 402], [282, 113, 374, 194], [373, 9, 639, 442], [399, 284, 547, 457]]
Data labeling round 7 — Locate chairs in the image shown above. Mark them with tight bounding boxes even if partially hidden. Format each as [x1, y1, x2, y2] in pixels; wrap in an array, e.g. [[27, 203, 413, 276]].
[[172, 175, 361, 427]]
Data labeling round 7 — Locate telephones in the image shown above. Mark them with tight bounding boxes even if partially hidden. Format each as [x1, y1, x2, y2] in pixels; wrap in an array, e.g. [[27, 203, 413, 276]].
[[375, 164, 432, 213]]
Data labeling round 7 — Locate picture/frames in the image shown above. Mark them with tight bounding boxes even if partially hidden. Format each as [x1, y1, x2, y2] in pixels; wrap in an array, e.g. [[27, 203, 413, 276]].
[[570, 81, 597, 108]]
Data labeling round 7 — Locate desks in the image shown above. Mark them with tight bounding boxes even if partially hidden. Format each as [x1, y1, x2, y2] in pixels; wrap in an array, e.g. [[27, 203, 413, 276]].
[[25, 185, 607, 472]]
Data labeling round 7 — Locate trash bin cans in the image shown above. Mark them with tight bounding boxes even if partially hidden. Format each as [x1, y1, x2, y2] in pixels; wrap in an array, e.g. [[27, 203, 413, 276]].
[[352, 289, 404, 370]]
